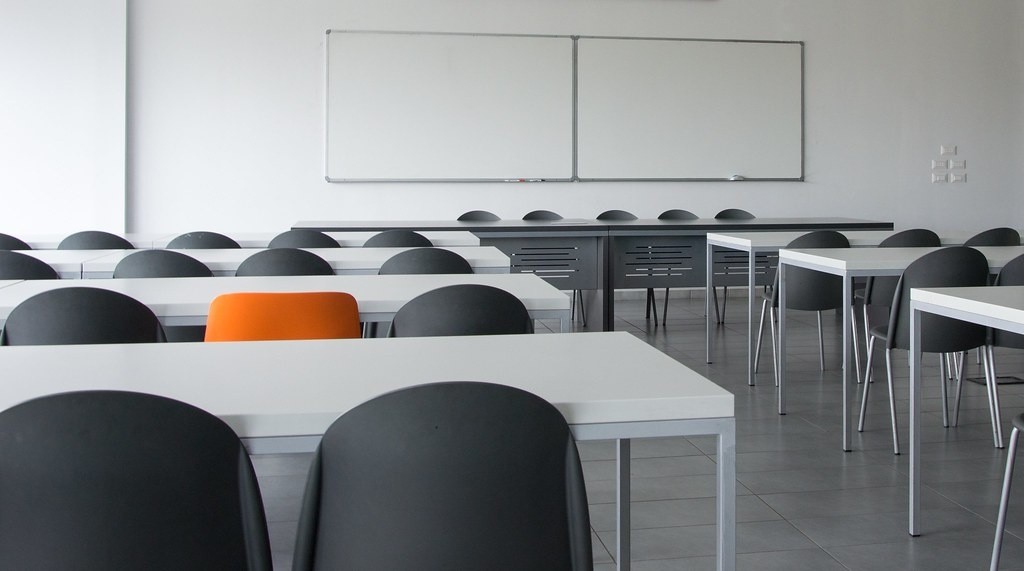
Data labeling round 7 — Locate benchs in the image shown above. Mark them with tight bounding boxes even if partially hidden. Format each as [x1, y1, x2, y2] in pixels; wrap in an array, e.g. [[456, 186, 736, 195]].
[[909, 285, 1024, 537]]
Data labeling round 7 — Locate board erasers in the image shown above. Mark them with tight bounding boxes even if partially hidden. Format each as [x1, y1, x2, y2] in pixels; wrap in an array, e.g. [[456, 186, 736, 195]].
[[730, 175, 745, 181]]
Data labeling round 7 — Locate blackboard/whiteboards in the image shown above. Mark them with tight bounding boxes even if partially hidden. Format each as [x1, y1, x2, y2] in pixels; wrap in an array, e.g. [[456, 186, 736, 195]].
[[575, 35, 804, 182], [325, 29, 575, 183]]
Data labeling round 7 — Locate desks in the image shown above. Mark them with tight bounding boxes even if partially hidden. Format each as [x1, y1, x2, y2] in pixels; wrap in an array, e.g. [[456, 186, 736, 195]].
[[82, 245, 513, 276], [0, 279, 22, 290], [777, 245, 1024, 453], [1, 274, 574, 333], [3, 248, 125, 279], [1, 334, 735, 570], [290, 214, 610, 346], [705, 231, 1024, 388], [152, 231, 480, 248], [591, 215, 894, 334], [8, 233, 172, 250]]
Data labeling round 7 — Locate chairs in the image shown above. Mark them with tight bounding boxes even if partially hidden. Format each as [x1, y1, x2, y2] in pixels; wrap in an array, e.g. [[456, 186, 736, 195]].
[[0, 209, 1023, 571]]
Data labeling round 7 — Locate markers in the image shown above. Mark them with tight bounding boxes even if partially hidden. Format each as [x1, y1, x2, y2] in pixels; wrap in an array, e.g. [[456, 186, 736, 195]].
[[526, 179, 545, 182], [505, 179, 525, 182]]
